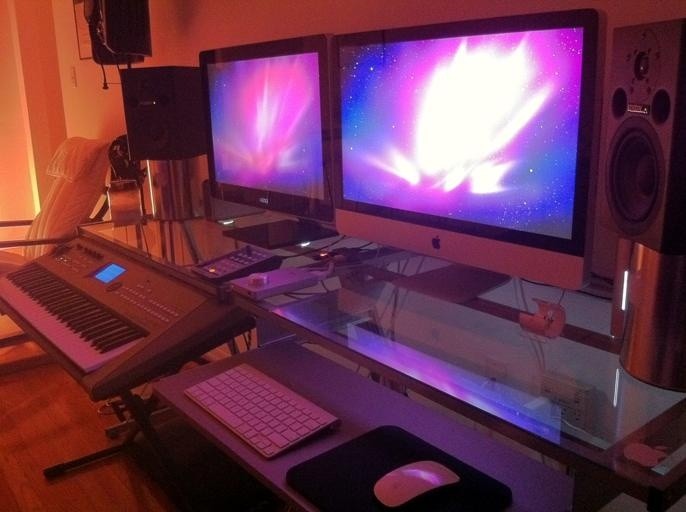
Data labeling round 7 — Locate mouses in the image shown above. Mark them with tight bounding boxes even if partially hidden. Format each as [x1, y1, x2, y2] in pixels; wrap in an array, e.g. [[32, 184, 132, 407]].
[[373, 460, 460, 507]]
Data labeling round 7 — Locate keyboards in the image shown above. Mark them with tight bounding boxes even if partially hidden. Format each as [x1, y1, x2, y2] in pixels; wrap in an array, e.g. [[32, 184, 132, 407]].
[[185, 364, 340, 460]]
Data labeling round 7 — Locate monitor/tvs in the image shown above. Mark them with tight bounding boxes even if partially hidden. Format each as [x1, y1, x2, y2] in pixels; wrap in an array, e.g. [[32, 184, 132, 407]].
[[199, 32, 330, 250], [330, 9, 607, 304]]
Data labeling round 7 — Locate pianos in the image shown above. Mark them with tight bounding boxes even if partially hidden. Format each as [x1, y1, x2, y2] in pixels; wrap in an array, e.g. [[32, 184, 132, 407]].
[[1, 236, 254, 401]]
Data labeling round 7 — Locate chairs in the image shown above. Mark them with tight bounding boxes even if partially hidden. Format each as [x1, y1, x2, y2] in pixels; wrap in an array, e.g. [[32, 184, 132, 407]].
[[1, 130, 121, 375]]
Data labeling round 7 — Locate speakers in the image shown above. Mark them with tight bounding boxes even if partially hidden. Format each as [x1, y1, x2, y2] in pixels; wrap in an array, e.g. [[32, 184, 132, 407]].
[[121, 66, 206, 161], [591, 19, 686, 284]]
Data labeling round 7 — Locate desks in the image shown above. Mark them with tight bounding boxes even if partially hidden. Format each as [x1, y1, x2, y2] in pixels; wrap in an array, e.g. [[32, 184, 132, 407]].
[[76, 210, 407, 302], [128, 247, 683, 510]]
